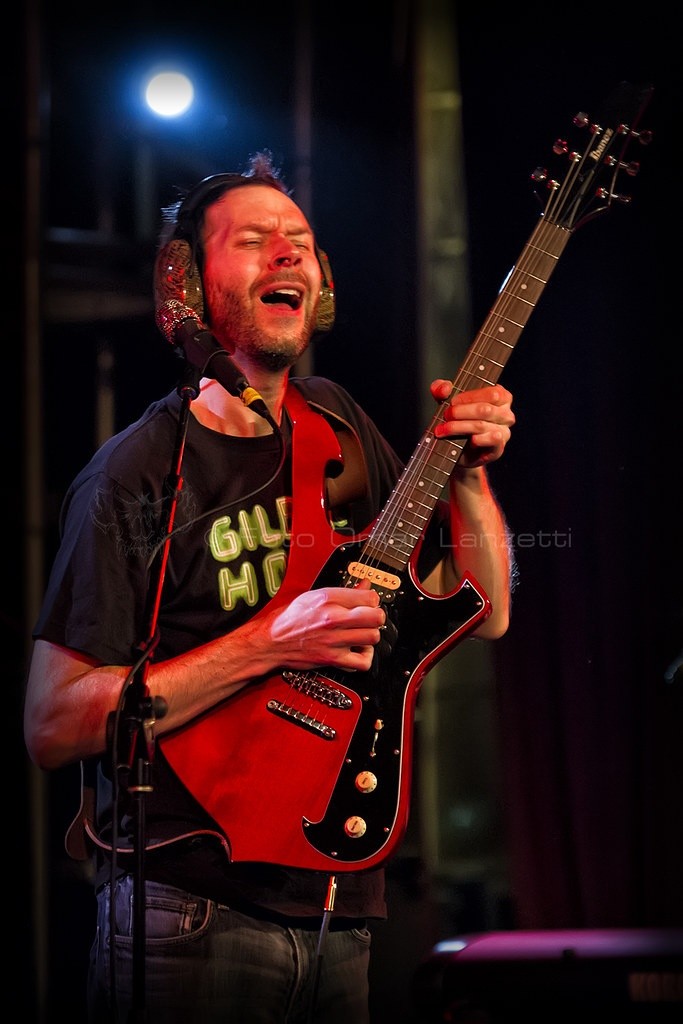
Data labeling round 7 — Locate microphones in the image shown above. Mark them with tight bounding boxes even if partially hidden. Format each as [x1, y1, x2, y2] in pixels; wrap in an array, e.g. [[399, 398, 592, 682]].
[[154, 299, 270, 419]]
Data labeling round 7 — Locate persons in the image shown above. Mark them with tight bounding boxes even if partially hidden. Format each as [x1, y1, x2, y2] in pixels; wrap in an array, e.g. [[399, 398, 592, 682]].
[[24, 154, 516, 1024]]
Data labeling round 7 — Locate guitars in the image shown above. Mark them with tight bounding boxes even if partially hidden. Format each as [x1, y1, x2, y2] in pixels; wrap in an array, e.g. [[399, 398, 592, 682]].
[[84, 106, 663, 874]]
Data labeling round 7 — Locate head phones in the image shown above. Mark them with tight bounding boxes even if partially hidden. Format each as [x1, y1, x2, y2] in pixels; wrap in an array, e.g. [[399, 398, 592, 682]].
[[152, 173, 334, 330]]
